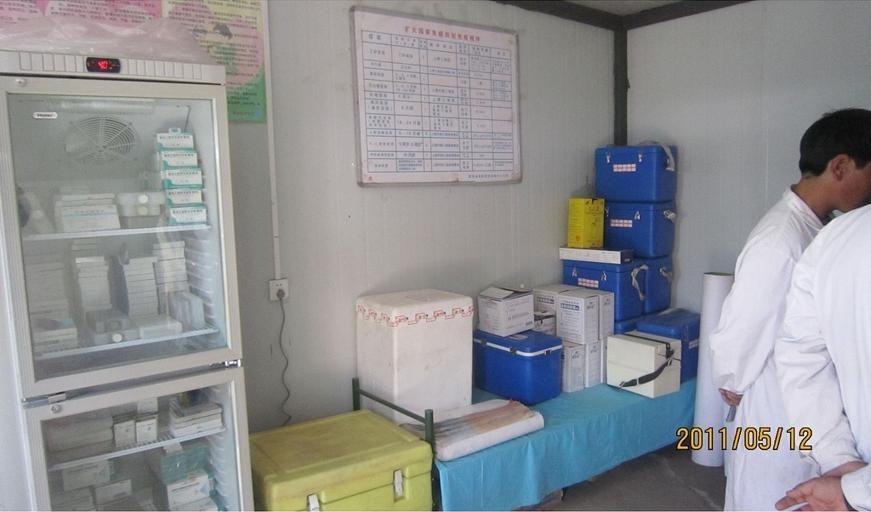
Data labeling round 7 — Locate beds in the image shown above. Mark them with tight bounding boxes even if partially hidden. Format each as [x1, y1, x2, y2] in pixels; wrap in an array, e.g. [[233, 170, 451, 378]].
[[352, 377, 697, 511]]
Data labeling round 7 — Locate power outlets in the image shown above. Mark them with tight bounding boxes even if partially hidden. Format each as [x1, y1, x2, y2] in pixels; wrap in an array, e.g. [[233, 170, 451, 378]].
[[269, 279, 288, 301]]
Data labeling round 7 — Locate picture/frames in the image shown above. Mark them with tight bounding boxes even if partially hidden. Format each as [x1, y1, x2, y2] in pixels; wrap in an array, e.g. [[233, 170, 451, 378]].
[[348, 5, 523, 189]]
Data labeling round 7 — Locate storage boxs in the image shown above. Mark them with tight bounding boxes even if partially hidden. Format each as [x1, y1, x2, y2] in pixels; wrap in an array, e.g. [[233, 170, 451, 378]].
[[558, 288, 614, 392], [563, 260, 642, 319], [642, 259, 673, 311], [607, 331, 682, 398], [637, 310, 698, 380], [355, 289, 472, 425], [477, 286, 535, 337], [594, 146, 678, 203], [567, 198, 603, 248], [472, 330, 564, 407], [605, 201, 673, 258], [250, 409, 435, 512]]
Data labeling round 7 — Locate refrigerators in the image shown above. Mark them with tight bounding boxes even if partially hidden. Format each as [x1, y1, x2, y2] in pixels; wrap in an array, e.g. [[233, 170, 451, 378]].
[[0, 47, 254, 512]]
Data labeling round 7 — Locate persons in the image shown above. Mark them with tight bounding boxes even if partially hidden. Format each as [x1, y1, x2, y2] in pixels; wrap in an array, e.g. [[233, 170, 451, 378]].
[[772, 201, 870, 511], [706, 107, 871, 511]]
